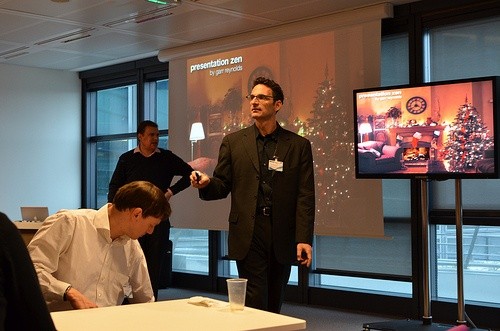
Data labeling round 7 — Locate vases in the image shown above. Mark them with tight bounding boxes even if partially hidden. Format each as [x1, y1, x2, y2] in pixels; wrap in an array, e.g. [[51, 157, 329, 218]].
[[392, 118, 397, 128]]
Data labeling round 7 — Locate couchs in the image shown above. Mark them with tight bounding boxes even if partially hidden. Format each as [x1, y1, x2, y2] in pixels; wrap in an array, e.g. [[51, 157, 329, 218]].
[[476, 150, 495, 174], [358, 141, 403, 174]]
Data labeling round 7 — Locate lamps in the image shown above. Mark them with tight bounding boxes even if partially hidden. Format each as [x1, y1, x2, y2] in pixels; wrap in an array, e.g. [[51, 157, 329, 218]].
[[359, 122, 372, 145], [189, 123, 205, 162]]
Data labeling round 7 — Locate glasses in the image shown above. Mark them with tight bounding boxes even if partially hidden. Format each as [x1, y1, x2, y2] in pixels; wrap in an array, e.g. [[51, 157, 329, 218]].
[[244, 94, 275, 100]]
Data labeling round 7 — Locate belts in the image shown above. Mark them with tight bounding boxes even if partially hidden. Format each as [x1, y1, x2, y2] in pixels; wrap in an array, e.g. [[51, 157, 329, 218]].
[[256, 207, 273, 217]]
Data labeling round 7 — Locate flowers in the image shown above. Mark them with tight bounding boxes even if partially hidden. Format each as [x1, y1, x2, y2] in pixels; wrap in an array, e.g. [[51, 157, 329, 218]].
[[385, 107, 402, 119]]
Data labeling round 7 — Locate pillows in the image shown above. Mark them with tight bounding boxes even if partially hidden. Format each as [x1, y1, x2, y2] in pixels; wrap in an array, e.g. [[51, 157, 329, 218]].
[[370, 147, 381, 158], [364, 150, 375, 159]]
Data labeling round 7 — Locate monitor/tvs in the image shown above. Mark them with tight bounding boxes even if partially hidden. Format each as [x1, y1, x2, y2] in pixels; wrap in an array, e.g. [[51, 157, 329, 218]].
[[352, 76, 500, 180], [19, 206, 48, 221]]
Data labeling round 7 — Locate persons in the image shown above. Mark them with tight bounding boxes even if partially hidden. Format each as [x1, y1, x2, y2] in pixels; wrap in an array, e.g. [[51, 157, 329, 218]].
[[107, 120, 195, 300], [190, 76, 315, 315], [27, 181, 172, 312]]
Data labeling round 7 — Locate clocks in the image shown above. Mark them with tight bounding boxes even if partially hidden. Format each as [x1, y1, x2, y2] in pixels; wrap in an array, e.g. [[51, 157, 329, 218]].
[[406, 96, 426, 115]]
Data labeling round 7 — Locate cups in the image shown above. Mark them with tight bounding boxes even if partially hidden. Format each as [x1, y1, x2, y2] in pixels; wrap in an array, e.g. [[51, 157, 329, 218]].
[[226, 278, 248, 310]]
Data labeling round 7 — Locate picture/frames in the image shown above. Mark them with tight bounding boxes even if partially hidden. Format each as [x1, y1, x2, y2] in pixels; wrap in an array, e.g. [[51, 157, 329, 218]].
[[206, 112, 222, 136], [373, 116, 386, 131]]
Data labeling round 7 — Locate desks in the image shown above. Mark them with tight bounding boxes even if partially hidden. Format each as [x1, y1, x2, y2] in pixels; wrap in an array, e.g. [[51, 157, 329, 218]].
[[15, 222, 42, 245], [48, 297, 306, 331]]
